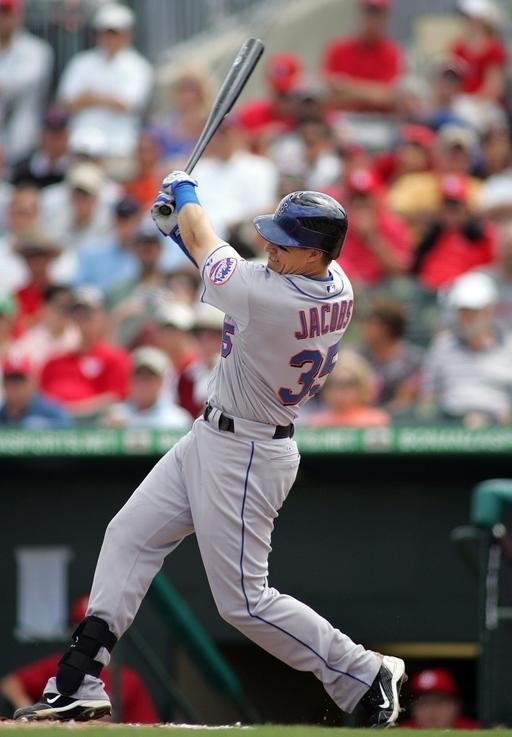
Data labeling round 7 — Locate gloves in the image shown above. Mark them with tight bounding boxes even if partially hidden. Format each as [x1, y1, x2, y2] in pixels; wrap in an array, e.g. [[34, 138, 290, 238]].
[[150, 171, 197, 243]]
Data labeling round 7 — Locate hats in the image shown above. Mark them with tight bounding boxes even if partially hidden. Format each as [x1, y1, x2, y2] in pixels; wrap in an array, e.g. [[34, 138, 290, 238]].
[[445, 273, 498, 310], [124, 344, 170, 376], [65, 284, 102, 310], [91, 2, 135, 32], [67, 161, 102, 195]]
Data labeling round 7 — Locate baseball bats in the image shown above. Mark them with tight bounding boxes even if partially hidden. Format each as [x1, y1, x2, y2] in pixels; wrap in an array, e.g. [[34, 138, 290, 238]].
[[161, 39, 264, 217]]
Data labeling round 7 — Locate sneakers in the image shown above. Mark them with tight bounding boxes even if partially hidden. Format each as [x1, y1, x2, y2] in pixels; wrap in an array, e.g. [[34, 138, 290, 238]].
[[365, 656, 407, 728], [13, 693, 111, 722]]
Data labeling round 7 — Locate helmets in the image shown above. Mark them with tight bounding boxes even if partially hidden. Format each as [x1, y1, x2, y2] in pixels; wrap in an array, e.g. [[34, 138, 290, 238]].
[[254, 191, 349, 260]]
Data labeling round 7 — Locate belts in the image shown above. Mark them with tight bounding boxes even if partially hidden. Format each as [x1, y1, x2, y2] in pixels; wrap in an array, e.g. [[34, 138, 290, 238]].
[[203, 402, 294, 438]]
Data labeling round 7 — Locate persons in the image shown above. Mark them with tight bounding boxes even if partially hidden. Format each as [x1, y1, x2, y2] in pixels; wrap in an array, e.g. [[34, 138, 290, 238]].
[[392, 670, 482, 729], [4, 595, 160, 724], [1, 1, 510, 429], [13, 168, 404, 730]]
